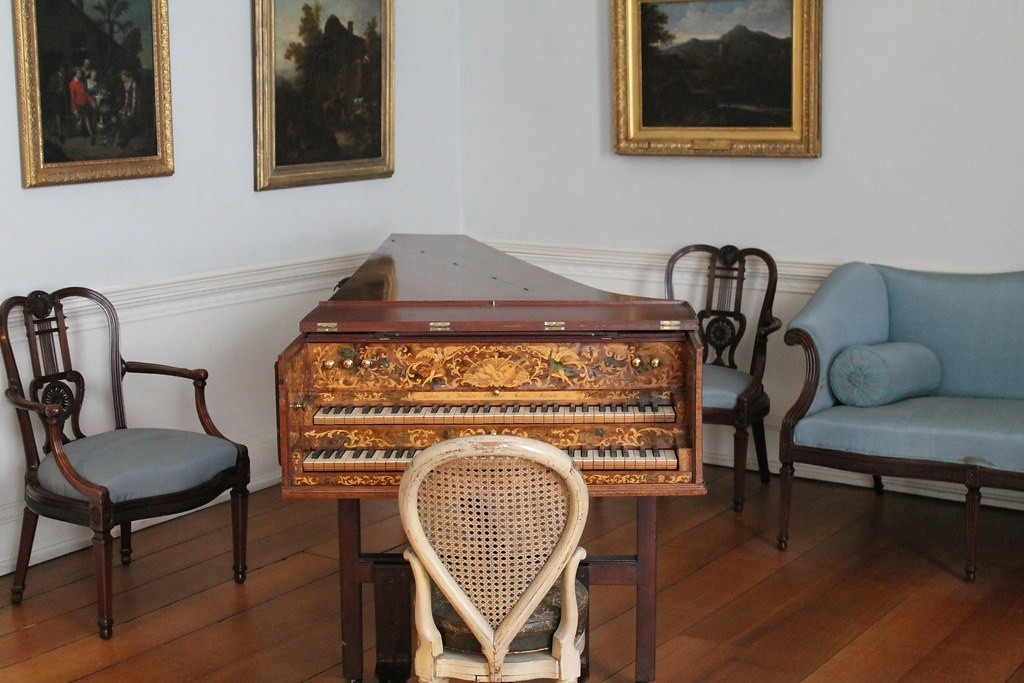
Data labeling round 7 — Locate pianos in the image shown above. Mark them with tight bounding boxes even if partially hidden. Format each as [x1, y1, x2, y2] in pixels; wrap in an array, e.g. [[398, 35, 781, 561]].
[[274, 232, 706, 683]]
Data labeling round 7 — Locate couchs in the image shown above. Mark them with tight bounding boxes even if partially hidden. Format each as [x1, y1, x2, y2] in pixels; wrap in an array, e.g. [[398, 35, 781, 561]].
[[775, 260, 1022, 572]]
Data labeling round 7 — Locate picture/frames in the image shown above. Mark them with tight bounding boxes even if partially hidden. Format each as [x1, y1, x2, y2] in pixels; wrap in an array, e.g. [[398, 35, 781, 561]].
[[242, 0, 396, 191], [12, 0, 176, 188], [609, 1, 823, 161]]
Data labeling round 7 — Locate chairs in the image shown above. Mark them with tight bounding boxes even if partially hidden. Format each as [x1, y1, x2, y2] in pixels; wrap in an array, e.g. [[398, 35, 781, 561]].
[[400, 436, 595, 683], [664, 244, 782, 511], [0, 286, 253, 639]]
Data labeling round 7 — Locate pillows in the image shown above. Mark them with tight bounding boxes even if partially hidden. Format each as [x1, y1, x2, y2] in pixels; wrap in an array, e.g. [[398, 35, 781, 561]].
[[827, 340, 941, 408]]
